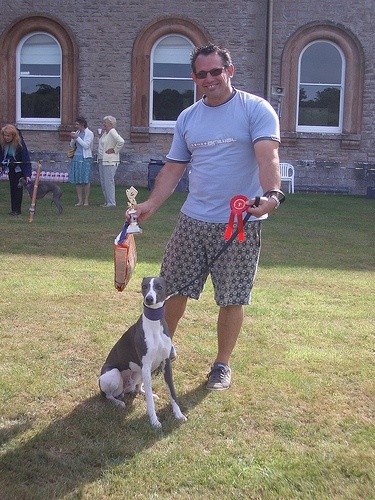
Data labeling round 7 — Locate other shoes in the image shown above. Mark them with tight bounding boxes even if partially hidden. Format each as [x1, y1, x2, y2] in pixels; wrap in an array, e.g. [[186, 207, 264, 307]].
[[100, 203, 106, 207], [104, 202, 116, 208], [84, 203, 88, 206], [9, 212, 14, 215], [75, 202, 83, 207], [13, 212, 19, 216]]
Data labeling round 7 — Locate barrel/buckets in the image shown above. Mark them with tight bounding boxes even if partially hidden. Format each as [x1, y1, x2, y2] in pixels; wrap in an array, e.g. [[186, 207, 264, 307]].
[[367, 186, 375, 198]]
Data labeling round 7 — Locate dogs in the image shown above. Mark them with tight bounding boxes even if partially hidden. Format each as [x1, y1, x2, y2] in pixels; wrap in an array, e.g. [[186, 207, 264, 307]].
[[99, 275, 189, 427], [17, 177, 63, 213]]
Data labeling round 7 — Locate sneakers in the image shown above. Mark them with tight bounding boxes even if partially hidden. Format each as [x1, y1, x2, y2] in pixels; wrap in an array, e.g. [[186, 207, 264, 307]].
[[170, 347, 177, 362], [206, 363, 231, 390]]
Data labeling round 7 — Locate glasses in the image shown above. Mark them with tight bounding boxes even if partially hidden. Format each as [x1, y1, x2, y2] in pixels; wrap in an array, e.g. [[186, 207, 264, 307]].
[[195, 65, 226, 78]]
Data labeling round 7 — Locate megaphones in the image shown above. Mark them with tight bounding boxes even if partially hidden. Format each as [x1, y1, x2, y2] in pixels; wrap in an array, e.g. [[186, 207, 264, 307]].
[[271, 85, 286, 96]]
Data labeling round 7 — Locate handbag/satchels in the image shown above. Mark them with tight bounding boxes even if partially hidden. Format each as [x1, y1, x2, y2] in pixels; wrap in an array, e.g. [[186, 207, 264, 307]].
[[114, 222, 137, 291], [67, 143, 76, 158]]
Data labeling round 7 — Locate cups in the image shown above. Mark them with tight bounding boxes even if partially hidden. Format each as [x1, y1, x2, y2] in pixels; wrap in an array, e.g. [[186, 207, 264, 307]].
[[98, 129, 102, 134]]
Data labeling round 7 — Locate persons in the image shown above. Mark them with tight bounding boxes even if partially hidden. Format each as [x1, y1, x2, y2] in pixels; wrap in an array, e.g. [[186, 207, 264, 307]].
[[0, 124, 32, 215], [125, 41, 281, 391], [70, 117, 94, 206], [97, 116, 125, 207]]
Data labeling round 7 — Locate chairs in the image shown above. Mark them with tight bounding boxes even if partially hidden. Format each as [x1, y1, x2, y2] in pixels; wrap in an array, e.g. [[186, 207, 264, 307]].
[[280, 163, 295, 194]]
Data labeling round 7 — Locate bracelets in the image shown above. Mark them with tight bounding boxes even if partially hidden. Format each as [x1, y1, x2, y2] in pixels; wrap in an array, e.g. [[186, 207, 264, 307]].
[[272, 195, 280, 209]]
